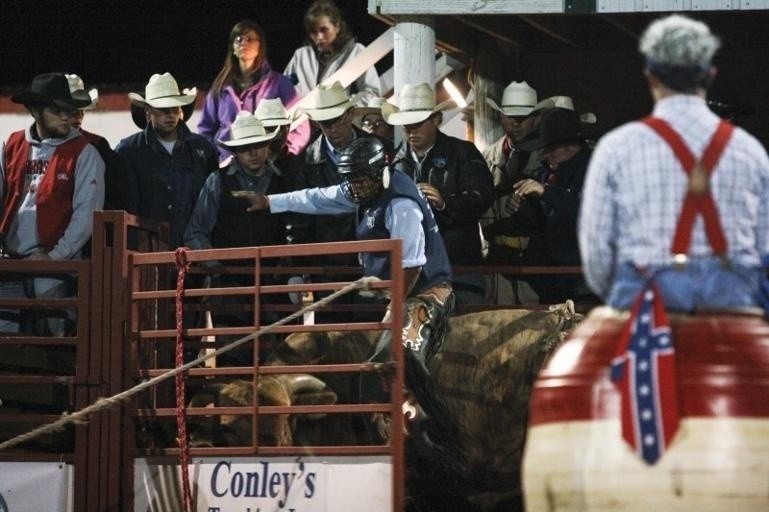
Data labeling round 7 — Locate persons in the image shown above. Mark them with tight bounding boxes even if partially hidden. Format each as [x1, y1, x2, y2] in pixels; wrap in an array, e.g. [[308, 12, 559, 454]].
[[575, 14, 768, 319], [280, 0, 383, 111], [194, 19, 312, 176], [0, 70, 608, 450]]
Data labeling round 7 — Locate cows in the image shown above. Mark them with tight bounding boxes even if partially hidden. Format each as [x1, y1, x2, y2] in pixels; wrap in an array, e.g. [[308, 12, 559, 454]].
[[179, 301, 588, 511]]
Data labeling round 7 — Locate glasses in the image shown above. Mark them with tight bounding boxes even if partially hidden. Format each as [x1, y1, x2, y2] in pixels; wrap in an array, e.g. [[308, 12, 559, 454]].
[[317, 112, 350, 130], [360, 119, 383, 128], [235, 35, 261, 43]]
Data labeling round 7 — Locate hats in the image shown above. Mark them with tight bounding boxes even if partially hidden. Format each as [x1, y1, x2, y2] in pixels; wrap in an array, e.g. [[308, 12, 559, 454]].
[[351, 97, 387, 130], [549, 96, 597, 124], [485, 80, 555, 116], [218, 80, 351, 145], [381, 82, 457, 126], [131, 93, 196, 130], [127, 72, 199, 109], [638, 14, 718, 70], [12, 75, 99, 112]]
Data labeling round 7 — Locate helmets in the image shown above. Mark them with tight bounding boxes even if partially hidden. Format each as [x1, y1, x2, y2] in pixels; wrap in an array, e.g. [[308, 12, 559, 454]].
[[338, 136, 393, 204]]
